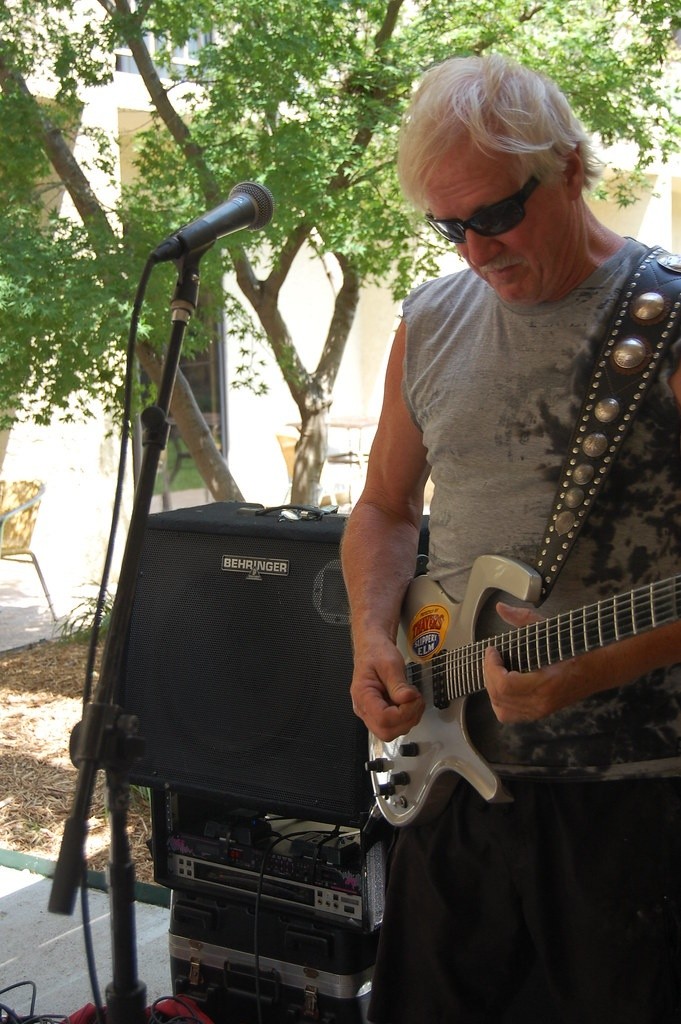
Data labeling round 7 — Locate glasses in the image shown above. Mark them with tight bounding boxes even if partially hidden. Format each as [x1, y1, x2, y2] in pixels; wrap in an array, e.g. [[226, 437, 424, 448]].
[[423, 176, 543, 243]]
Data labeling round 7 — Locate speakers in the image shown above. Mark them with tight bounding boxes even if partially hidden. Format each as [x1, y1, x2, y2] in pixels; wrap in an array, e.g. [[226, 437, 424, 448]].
[[121, 500, 434, 829]]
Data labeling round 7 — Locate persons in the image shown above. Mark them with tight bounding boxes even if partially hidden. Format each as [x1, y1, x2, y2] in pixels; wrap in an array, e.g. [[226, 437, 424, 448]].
[[338, 54, 681, 1024]]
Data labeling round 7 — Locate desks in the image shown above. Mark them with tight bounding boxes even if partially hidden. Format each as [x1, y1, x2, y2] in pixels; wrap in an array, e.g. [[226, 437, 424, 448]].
[[284, 416, 382, 512]]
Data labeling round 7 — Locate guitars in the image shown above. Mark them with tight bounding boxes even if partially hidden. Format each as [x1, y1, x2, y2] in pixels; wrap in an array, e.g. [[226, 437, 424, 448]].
[[363, 551, 681, 824]]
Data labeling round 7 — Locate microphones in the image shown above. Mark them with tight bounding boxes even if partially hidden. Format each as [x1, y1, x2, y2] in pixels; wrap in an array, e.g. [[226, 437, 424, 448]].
[[151, 182, 276, 265]]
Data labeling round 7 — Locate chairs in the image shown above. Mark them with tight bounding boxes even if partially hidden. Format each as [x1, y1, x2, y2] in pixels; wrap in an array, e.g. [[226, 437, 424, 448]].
[[0, 479, 60, 620], [278, 435, 299, 507]]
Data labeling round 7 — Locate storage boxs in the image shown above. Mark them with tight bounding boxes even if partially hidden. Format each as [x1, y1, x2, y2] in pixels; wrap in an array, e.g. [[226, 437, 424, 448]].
[[168, 900, 380, 1024]]
